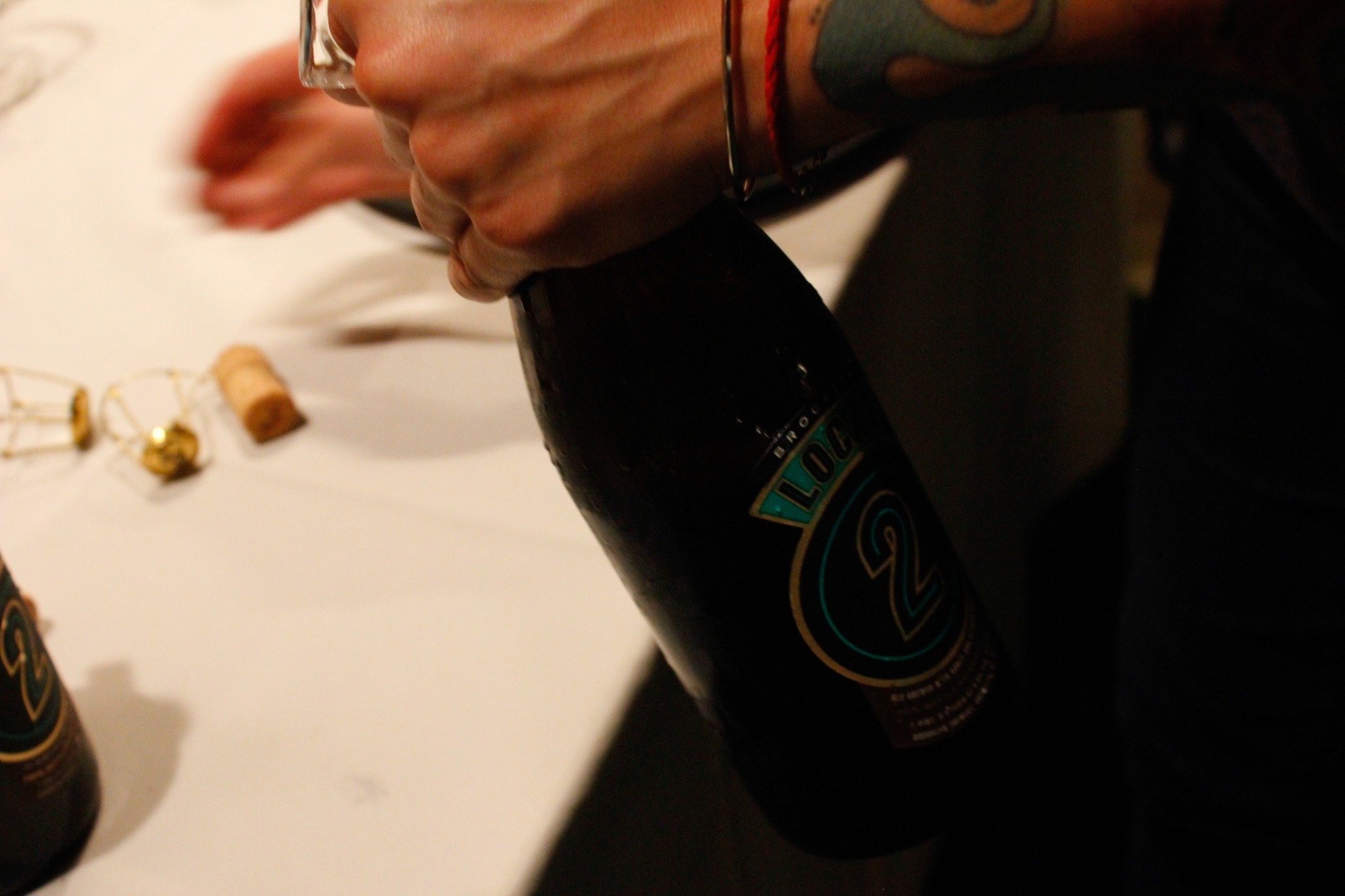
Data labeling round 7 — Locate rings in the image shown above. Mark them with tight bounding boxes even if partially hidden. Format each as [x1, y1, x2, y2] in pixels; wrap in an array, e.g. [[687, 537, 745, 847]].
[[296, 0, 357, 93]]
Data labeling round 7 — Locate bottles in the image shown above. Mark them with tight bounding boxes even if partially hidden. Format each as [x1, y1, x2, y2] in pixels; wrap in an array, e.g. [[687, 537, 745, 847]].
[[510, 219, 1032, 863], [0, 551, 100, 896]]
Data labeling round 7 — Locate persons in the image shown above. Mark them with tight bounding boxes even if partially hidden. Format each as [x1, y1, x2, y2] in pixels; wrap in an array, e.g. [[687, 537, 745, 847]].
[[191, 0, 1345, 891]]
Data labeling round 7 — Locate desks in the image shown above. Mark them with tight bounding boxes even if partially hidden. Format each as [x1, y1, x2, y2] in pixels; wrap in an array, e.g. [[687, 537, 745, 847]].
[[0, 0, 907, 891]]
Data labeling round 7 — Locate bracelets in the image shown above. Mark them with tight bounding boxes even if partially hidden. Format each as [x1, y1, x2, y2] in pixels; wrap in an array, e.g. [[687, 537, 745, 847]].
[[766, 0, 820, 199], [718, 0, 754, 206]]
